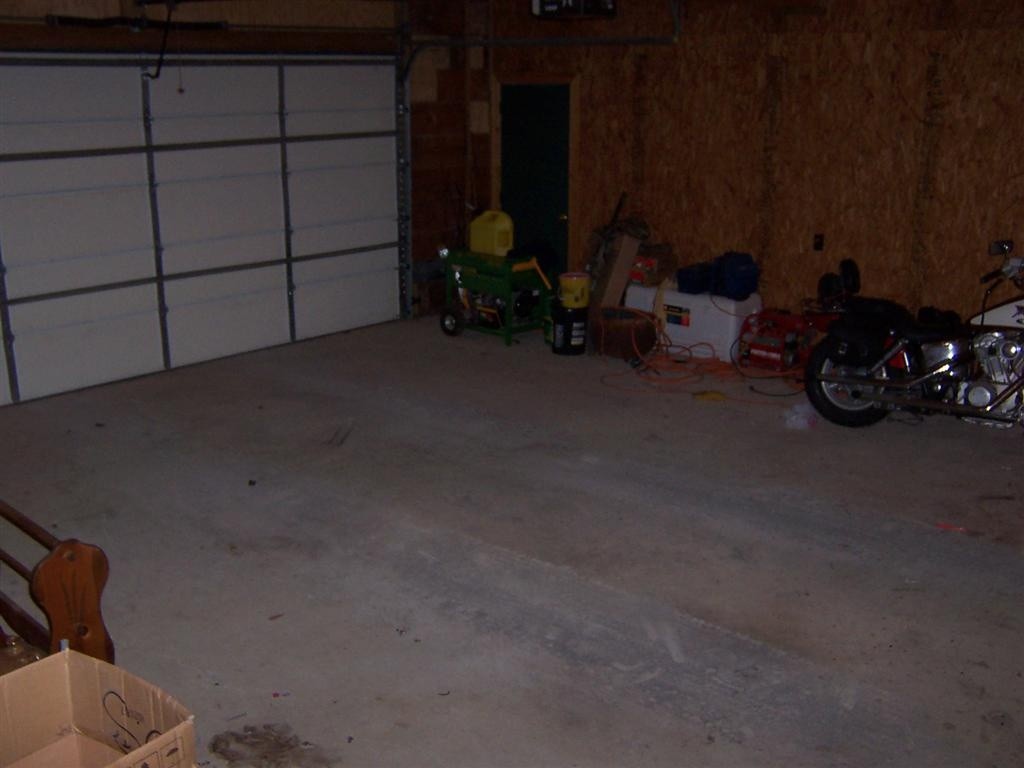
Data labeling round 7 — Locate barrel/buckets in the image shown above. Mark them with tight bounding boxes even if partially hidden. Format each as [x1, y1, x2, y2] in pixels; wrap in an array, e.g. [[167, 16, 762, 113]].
[[550, 296, 587, 356], [559, 271, 589, 308]]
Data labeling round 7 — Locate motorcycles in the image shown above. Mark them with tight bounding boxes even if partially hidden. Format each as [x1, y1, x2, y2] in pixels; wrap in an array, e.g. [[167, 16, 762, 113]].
[[805, 239, 1024, 427]]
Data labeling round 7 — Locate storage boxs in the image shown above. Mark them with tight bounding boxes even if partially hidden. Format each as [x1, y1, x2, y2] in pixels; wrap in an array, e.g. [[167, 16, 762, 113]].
[[0, 650, 197, 768], [590, 231, 763, 365]]
